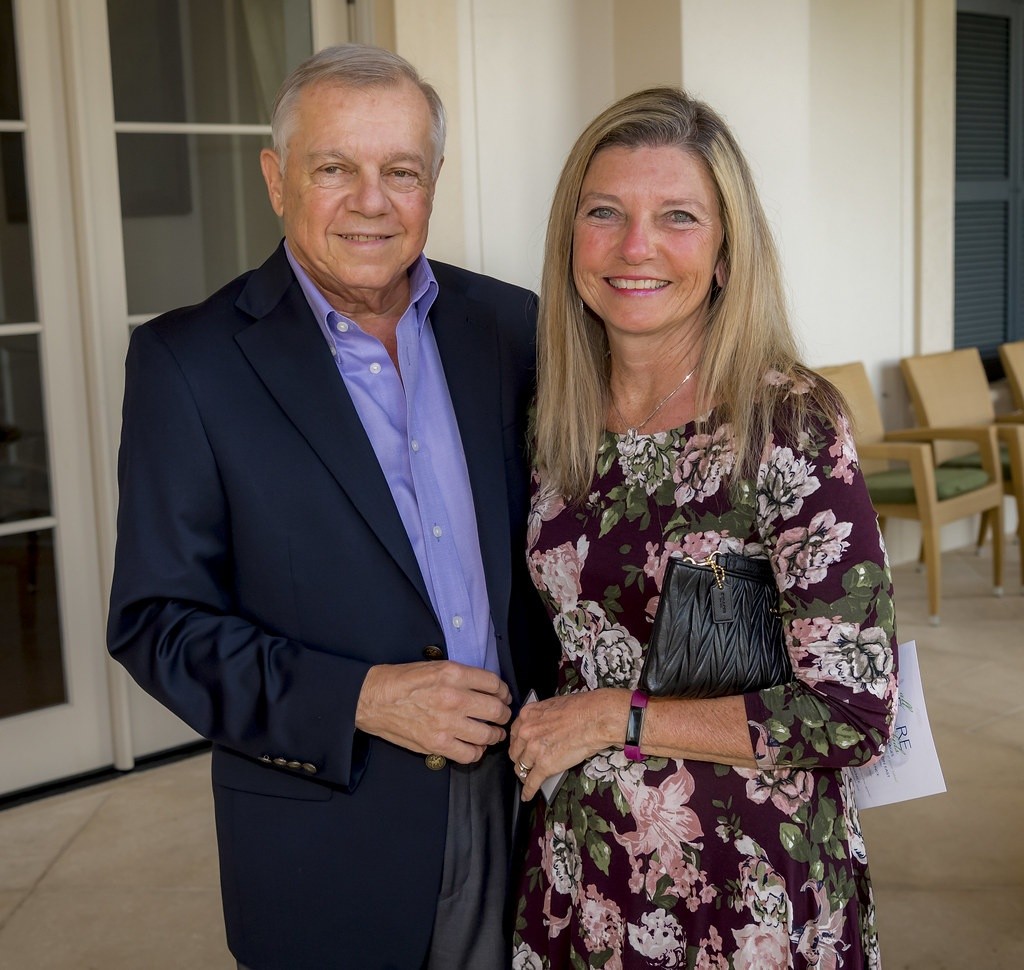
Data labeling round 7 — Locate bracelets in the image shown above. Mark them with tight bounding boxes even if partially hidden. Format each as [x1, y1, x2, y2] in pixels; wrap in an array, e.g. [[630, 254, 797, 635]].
[[624, 691, 649, 762]]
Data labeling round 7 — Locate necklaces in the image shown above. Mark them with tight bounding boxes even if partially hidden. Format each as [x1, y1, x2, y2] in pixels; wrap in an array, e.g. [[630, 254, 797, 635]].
[[604, 358, 704, 457]]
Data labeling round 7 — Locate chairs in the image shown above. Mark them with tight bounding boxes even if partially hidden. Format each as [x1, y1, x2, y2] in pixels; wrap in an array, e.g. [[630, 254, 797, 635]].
[[809, 361, 1004, 625], [975, 343, 1024, 550], [897, 347, 1022, 584]]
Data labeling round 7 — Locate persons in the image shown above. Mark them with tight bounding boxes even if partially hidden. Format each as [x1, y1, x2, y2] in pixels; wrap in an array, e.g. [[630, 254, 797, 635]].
[[104, 41, 542, 967], [510, 86, 901, 970]]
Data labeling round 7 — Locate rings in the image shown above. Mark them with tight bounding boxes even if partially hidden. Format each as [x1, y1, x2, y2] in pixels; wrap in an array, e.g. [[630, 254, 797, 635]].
[[516, 759, 532, 778]]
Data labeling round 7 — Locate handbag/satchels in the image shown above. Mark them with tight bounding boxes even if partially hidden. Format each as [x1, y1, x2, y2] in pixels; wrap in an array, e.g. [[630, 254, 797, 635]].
[[637, 551, 793, 701]]
